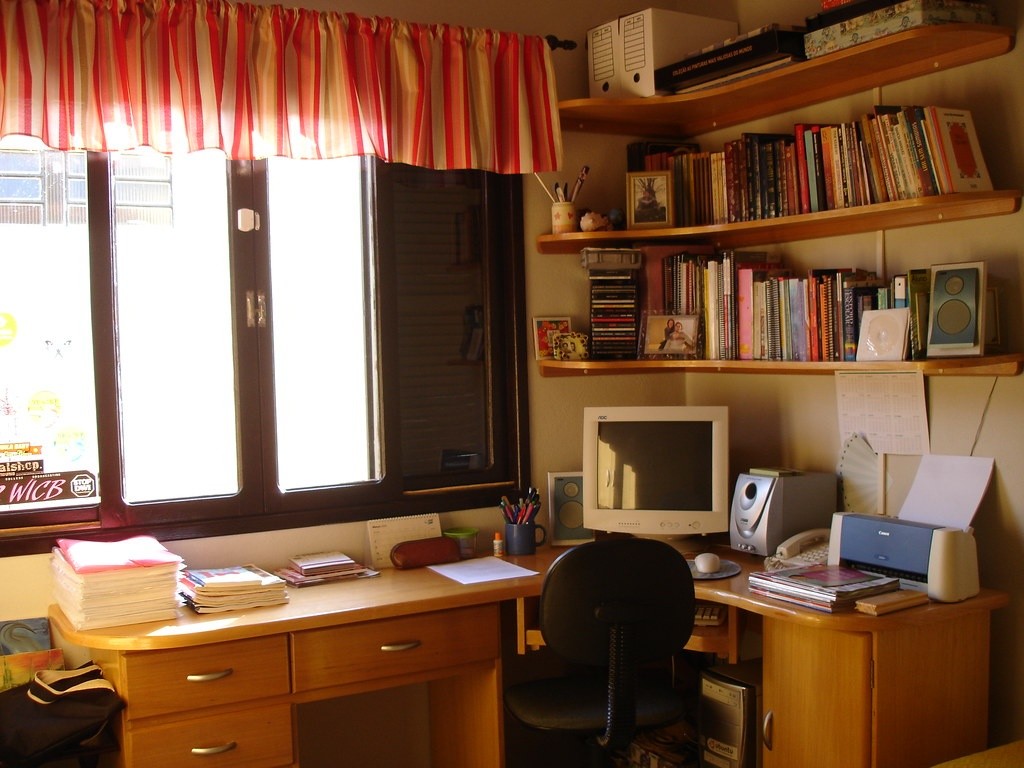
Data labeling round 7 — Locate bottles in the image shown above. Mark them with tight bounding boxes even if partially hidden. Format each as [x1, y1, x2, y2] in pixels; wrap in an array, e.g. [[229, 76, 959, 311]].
[[493, 532, 505, 557]]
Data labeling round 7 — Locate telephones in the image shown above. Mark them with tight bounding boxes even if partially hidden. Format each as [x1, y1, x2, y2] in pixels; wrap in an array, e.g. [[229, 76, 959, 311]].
[[771, 528, 831, 567]]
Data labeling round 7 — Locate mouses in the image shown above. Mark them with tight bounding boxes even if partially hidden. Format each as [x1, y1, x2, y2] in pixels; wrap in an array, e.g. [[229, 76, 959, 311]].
[[695, 554, 720, 573]]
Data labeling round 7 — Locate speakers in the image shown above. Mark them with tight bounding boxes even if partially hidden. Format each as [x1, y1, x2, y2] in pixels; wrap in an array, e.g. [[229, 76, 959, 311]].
[[927, 260, 989, 357], [730, 472, 837, 558], [548, 472, 595, 546]]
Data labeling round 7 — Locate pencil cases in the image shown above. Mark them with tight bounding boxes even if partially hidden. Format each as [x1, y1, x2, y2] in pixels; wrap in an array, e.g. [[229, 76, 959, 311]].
[[390, 537, 460, 570]]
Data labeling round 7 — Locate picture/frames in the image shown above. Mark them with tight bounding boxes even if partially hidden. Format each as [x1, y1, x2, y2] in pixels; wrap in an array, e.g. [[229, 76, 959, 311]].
[[532, 316, 572, 360], [626, 172, 673, 231], [636, 308, 706, 361]]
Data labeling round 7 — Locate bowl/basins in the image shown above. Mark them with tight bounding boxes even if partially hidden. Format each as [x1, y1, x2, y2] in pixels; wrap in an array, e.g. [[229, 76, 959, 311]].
[[442, 527, 479, 558]]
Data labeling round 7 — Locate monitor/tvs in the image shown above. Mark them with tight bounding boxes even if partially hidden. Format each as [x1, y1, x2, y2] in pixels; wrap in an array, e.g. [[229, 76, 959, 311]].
[[582, 407, 730, 554]]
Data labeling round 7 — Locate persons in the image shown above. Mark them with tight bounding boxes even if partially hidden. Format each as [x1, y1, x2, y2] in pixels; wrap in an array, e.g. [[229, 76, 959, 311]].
[[637, 190, 658, 222], [562, 342, 580, 360], [657, 319, 694, 353]]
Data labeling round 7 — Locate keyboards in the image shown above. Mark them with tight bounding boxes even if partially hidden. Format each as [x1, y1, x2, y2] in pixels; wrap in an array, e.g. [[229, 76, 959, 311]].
[[694, 604, 726, 627]]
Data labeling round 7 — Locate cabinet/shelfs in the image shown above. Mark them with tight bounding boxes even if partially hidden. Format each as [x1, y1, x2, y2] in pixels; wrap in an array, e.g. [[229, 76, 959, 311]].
[[48, 535, 1011, 768], [537, 24, 1024, 378]]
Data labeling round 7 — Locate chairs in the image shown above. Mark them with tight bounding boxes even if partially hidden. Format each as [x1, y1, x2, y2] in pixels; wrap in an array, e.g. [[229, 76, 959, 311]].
[[503, 539, 696, 768]]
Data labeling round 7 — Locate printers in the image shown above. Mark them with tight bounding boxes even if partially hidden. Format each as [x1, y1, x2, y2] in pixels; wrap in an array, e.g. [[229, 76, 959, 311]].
[[827, 511, 980, 603]]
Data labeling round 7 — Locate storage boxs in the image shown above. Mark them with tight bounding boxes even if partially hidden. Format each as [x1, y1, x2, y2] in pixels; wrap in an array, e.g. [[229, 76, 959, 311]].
[[587, 8, 738, 100]]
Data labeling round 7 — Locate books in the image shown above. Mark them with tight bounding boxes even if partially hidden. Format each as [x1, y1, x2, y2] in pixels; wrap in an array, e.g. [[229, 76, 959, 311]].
[[748, 563, 900, 613], [661, 250, 930, 362], [626, 105, 995, 227], [178, 563, 289, 613], [272, 551, 381, 587], [590, 275, 637, 360]]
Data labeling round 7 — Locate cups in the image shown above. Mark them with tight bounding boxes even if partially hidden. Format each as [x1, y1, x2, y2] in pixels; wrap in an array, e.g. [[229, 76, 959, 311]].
[[551, 202, 576, 234], [505, 521, 546, 555]]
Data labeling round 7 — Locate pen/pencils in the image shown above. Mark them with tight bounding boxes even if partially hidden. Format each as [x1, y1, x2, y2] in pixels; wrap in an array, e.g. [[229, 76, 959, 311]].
[[533, 166, 592, 203], [500, 486, 542, 525]]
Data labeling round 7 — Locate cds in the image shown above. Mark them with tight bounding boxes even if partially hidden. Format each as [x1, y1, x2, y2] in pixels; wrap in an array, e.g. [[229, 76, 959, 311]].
[[868, 315, 899, 353]]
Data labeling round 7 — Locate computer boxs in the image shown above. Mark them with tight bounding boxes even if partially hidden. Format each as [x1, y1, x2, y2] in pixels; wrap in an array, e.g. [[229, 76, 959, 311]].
[[698, 670, 757, 768]]
